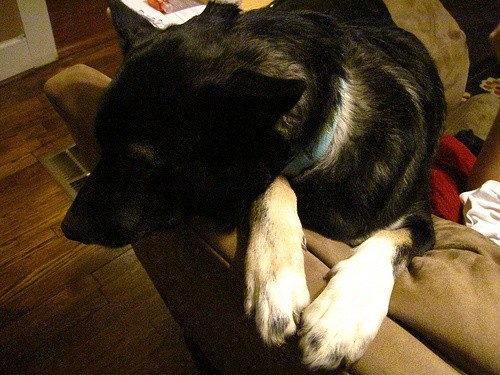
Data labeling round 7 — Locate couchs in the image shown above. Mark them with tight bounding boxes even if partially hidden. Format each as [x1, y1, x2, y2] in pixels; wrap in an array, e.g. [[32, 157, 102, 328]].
[[43, 0, 500, 375]]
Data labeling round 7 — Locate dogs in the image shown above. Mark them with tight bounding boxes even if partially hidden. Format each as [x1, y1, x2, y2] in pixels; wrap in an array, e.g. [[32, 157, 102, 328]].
[[60, 0, 447, 369]]
